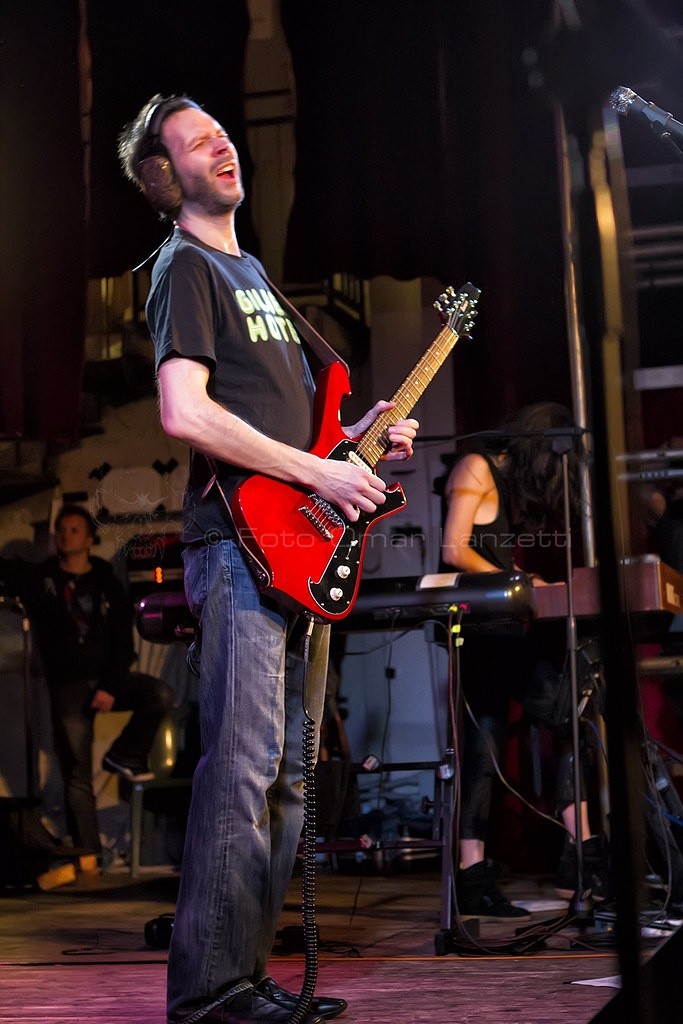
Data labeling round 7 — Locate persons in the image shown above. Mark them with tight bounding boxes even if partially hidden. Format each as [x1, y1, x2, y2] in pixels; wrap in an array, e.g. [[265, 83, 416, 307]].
[[0, 504, 178, 894], [438, 400, 617, 914], [118, 88, 418, 1024]]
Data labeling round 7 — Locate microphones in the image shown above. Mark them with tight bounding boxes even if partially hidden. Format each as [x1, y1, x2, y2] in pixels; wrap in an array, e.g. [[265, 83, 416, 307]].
[[609, 86, 683, 140]]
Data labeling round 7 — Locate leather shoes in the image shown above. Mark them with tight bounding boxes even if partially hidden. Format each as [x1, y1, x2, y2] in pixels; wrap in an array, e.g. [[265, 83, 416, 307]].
[[255, 977, 347, 1020], [190, 983, 325, 1024]]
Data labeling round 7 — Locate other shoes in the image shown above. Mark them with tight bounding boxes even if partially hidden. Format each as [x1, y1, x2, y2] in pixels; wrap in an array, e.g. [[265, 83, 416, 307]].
[[102, 748, 156, 782]]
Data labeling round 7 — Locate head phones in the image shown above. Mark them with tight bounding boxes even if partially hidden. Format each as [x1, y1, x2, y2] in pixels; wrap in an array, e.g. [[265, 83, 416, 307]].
[[138, 98, 202, 211]]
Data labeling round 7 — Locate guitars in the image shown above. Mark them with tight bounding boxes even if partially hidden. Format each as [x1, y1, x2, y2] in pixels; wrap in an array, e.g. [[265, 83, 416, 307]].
[[228, 279, 485, 626]]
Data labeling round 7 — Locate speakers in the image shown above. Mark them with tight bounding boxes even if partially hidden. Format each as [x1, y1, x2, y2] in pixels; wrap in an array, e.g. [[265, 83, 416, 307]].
[[587, 922, 683, 1024]]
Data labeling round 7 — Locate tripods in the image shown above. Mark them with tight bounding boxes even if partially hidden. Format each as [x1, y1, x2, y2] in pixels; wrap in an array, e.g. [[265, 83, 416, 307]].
[[406, 427, 678, 958]]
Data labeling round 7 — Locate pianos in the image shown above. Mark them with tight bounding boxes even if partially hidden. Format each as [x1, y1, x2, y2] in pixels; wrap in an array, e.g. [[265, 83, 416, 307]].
[[132, 568, 540, 647], [532, 554, 682, 623]]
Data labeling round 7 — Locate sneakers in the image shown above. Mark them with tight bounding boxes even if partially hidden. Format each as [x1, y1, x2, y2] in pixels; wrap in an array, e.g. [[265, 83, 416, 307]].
[[452, 860, 533, 922], [554, 834, 616, 902]]
